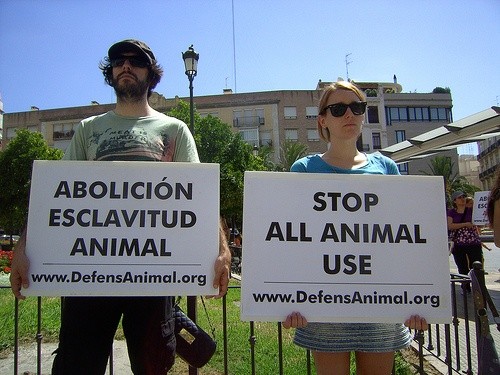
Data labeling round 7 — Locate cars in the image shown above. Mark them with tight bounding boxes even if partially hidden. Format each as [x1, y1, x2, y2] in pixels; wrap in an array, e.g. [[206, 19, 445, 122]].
[[229, 227, 240, 237], [0, 234, 21, 242]]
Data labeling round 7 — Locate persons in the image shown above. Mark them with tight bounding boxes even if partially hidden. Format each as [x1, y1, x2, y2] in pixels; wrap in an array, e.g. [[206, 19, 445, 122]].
[[282, 82, 428, 375], [232, 237, 242, 256], [11, 39, 231, 375], [447, 191, 483, 295]]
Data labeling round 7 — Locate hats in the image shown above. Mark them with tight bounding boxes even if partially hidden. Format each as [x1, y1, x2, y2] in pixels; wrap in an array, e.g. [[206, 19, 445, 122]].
[[108, 39, 155, 67], [451, 191, 467, 202]]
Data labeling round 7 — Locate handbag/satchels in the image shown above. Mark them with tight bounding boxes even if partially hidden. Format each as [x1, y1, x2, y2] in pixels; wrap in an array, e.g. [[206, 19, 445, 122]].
[[457, 227, 480, 244], [171, 295, 218, 368]]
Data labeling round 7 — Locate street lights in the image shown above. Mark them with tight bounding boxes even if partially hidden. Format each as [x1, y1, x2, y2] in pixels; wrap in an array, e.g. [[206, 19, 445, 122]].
[[181, 47, 199, 135]]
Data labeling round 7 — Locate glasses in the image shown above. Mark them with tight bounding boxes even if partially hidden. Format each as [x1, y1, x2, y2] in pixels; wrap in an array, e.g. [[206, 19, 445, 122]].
[[324, 101, 368, 117], [111, 55, 149, 67]]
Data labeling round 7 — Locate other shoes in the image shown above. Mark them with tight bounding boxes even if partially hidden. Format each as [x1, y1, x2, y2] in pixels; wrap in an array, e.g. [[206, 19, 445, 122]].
[[461, 289, 469, 295], [484, 270, 488, 274]]
